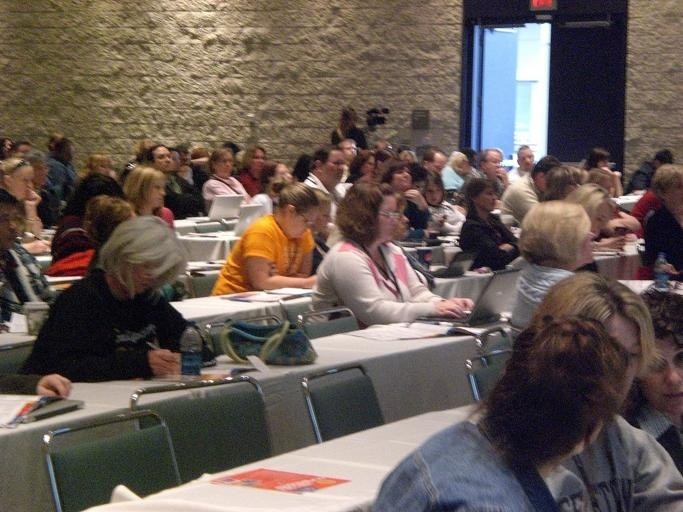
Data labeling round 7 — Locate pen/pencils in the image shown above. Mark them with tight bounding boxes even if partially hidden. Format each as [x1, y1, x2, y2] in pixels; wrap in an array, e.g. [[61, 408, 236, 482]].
[[147, 341, 161, 351]]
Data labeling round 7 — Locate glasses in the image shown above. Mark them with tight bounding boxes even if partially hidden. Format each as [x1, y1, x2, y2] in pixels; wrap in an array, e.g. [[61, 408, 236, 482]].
[[121, 162, 136, 181]]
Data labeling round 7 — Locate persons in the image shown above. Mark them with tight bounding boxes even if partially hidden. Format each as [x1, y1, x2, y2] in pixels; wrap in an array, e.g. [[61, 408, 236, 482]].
[[365, 309, 636, 511], [619, 286, 682, 477], [2, 106, 681, 401], [517, 267, 683, 511]]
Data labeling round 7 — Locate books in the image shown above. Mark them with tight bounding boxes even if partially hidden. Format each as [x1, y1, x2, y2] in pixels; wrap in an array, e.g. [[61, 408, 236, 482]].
[[0, 394, 61, 430]]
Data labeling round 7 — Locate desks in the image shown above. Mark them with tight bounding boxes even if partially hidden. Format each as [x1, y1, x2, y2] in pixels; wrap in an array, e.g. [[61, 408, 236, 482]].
[[1, 319, 520, 512], [22, 183, 514, 337]]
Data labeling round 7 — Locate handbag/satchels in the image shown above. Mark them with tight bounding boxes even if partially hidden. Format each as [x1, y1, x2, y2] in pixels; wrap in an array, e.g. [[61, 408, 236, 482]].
[[219, 318, 317, 365]]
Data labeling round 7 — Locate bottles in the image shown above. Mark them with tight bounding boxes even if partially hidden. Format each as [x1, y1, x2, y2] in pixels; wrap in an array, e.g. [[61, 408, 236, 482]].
[[177, 322, 204, 382], [653, 250, 669, 294]]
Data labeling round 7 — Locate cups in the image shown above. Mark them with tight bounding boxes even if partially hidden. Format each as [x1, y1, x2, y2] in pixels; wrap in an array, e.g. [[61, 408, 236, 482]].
[[442, 246, 464, 266], [415, 245, 432, 268]]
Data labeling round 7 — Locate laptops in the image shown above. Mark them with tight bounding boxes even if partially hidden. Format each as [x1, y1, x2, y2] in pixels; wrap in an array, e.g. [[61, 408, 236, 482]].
[[427, 251, 479, 277], [186, 195, 244, 222], [418, 269, 522, 323], [199, 203, 265, 237]]
